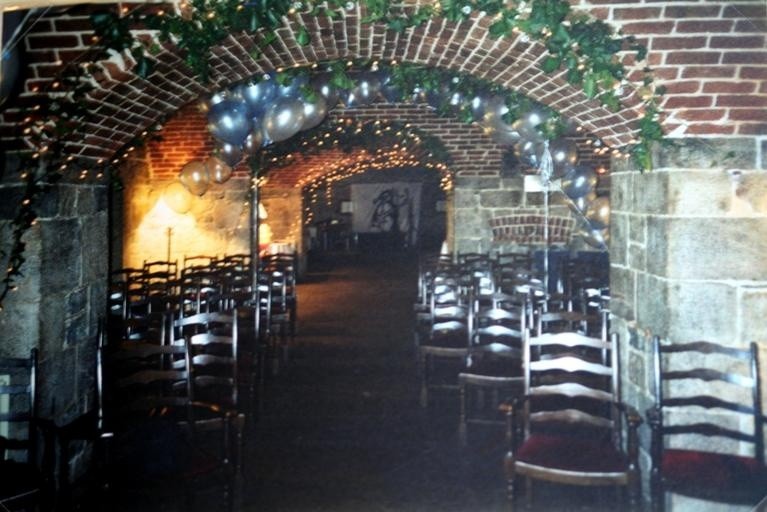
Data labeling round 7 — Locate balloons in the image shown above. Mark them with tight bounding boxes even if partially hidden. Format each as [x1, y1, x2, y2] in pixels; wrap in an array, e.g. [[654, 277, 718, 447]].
[[541, 137, 610, 258], [195, 69, 565, 167], [164, 154, 232, 215]]
